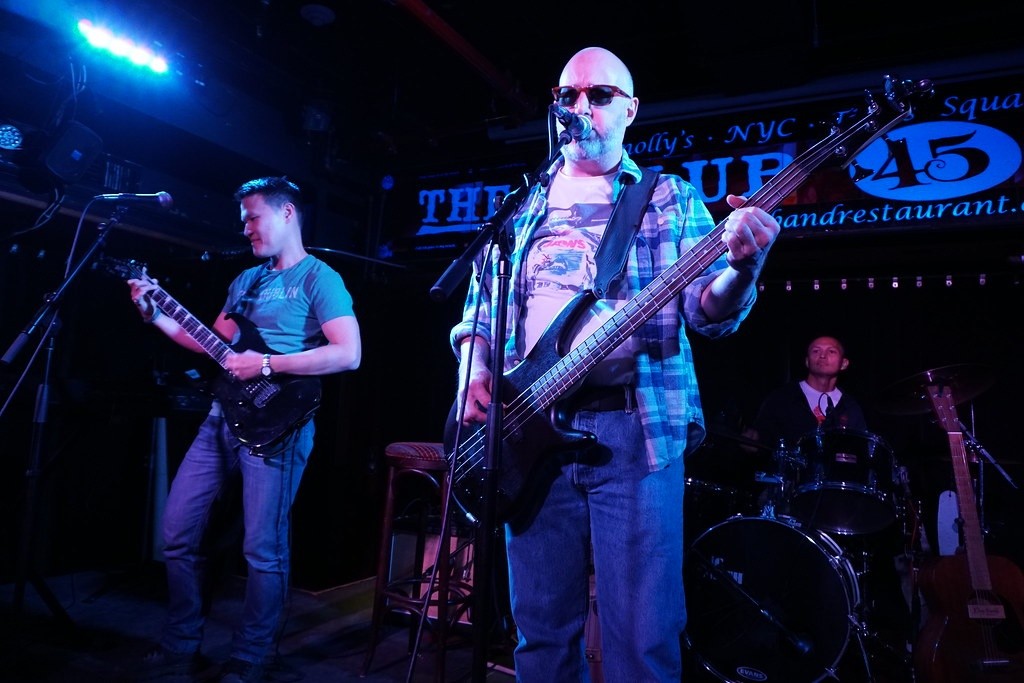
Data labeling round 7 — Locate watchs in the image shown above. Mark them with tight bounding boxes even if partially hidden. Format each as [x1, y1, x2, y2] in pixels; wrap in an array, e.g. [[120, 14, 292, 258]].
[[261, 354, 273, 380]]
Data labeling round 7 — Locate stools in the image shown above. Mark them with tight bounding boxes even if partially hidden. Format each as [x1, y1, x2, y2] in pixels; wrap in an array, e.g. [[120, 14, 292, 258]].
[[360, 441, 487, 683]]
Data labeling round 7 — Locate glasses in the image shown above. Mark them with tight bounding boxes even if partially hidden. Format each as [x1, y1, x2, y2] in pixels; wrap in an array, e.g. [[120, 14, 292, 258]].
[[553, 84, 630, 107]]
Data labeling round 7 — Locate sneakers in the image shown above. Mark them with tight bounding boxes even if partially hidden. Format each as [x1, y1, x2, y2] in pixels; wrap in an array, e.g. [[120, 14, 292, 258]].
[[216, 657, 272, 683], [119, 644, 203, 683]]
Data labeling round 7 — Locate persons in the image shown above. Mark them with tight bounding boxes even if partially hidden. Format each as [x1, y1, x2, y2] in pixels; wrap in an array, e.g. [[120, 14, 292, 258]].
[[763, 335, 863, 456], [449, 47, 781, 683], [126, 176, 361, 683]]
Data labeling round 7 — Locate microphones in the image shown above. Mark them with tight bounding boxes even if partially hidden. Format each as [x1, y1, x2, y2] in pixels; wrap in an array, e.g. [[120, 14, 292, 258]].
[[827, 396, 833, 407], [93, 191, 172, 209], [550, 103, 592, 141], [793, 641, 811, 655]]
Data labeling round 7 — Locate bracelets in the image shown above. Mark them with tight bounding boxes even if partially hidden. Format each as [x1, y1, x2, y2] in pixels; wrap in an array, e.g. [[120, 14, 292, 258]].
[[144, 308, 160, 323]]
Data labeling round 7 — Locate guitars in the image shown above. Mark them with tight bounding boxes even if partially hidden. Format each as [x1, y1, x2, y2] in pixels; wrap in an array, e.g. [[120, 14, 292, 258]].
[[916, 387, 1023, 683], [102, 254, 325, 454], [440, 69, 936, 532]]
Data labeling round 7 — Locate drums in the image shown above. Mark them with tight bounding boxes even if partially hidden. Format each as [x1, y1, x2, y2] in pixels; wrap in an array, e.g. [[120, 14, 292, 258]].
[[784, 423, 906, 538], [677, 509, 860, 682], [682, 478, 736, 547]]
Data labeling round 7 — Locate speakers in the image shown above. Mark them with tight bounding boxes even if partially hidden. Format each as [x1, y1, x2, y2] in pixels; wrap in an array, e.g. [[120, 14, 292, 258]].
[[44, 122, 102, 184], [383, 512, 475, 633]]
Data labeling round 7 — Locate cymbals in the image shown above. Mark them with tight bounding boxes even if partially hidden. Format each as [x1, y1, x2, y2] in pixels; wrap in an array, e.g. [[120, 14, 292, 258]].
[[875, 359, 994, 420]]
